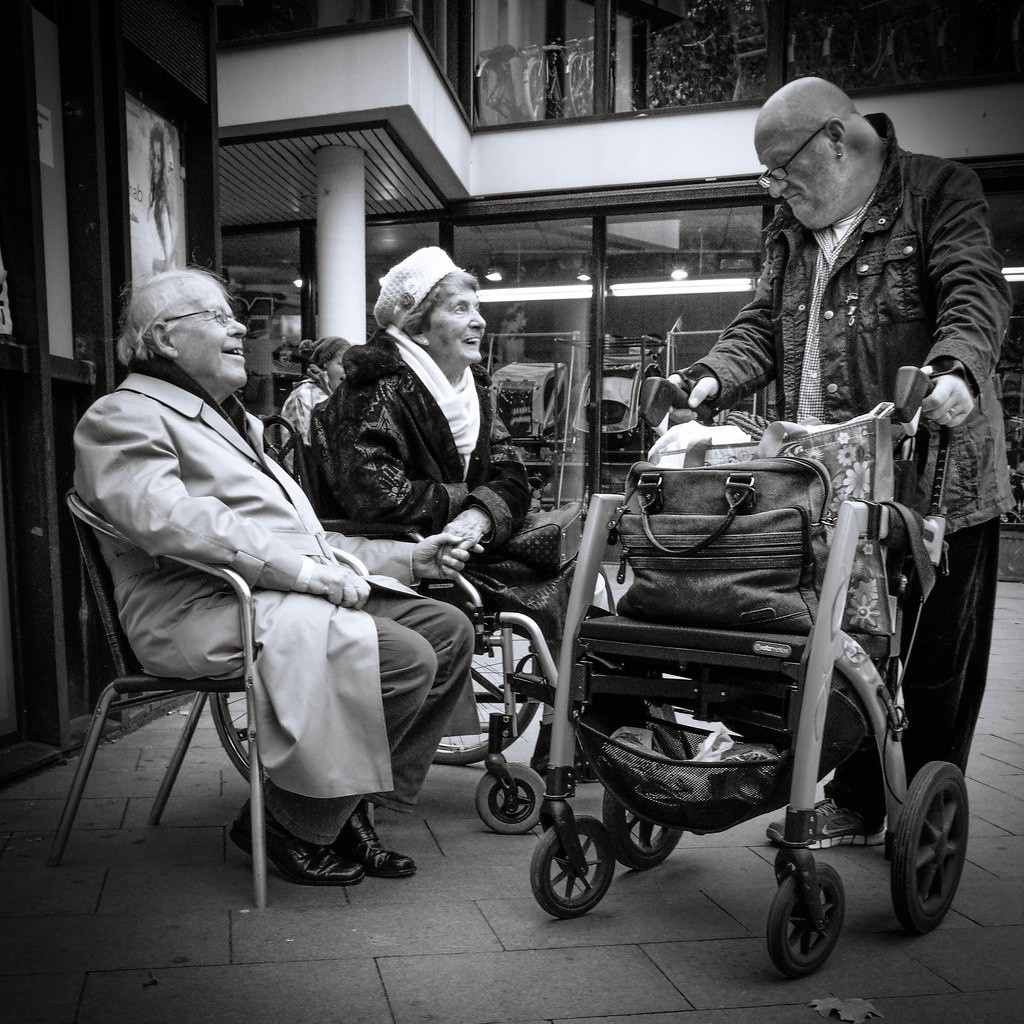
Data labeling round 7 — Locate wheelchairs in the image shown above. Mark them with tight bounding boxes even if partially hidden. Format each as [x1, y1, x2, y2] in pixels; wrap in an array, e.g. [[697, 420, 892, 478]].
[[261, 415, 618, 836]]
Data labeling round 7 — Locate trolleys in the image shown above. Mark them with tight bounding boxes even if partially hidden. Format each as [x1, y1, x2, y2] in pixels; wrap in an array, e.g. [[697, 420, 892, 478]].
[[529, 375, 970, 979]]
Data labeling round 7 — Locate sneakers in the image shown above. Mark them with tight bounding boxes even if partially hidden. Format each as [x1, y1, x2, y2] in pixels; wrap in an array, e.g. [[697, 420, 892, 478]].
[[765, 799, 888, 849]]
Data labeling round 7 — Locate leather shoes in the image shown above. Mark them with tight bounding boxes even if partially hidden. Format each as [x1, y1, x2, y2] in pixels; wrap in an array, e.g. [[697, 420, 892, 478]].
[[334, 810, 417, 877], [230, 798, 365, 886]]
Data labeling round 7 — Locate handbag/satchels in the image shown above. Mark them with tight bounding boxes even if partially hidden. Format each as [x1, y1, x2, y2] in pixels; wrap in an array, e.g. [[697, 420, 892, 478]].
[[500, 501, 583, 573], [605, 460, 831, 635], [643, 402, 897, 637]]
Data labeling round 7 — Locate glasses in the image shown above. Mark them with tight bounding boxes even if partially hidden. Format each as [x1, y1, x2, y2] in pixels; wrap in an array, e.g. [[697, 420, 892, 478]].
[[162, 305, 236, 327], [755, 121, 831, 188]]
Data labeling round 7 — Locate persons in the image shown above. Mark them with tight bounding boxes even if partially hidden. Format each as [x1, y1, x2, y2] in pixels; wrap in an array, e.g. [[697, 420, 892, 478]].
[[307, 247, 587, 776], [70, 264, 491, 886], [668, 74, 1024, 854], [279, 335, 351, 479], [497, 301, 525, 368], [143, 121, 178, 277]]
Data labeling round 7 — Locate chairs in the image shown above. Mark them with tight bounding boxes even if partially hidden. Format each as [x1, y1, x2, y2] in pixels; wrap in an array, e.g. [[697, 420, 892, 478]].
[[45, 489, 369, 910]]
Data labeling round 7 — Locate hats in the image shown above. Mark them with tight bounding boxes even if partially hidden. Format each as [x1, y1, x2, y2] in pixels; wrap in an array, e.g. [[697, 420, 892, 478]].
[[299, 337, 350, 373], [374, 246, 462, 328]]
